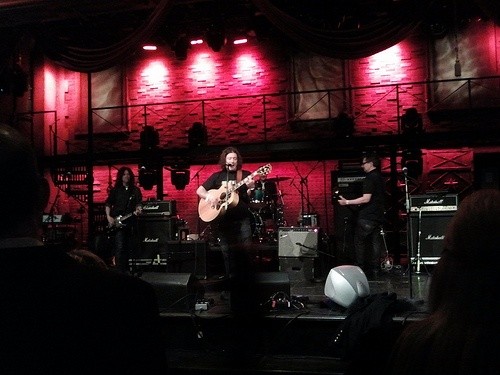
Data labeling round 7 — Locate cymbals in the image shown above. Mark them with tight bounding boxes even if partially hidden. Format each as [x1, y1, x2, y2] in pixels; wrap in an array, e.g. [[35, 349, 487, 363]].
[[272, 193, 287, 196], [264, 177, 291, 181]]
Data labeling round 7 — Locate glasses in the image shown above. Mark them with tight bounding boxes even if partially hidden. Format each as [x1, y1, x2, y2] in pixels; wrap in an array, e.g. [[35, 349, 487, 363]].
[[361, 161, 367, 164]]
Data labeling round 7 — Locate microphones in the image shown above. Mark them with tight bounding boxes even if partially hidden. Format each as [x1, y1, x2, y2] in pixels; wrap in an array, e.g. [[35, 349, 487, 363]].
[[226, 163, 234, 166], [402, 167, 407, 175]]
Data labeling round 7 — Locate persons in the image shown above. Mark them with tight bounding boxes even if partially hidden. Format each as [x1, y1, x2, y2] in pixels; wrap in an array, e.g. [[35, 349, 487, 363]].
[[427, 153, 500, 307], [342, 291, 500, 375], [196, 147, 256, 280], [103, 167, 143, 267], [337, 151, 385, 282], [0, 122, 169, 375]]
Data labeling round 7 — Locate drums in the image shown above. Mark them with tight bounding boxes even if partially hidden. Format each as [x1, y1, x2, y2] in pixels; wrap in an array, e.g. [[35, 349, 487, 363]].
[[300, 213, 319, 228], [248, 210, 264, 239], [247, 189, 268, 208]]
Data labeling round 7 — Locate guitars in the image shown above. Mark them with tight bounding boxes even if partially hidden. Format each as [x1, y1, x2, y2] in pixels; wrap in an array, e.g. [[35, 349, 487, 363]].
[[104, 203, 159, 236], [198, 163, 272, 222]]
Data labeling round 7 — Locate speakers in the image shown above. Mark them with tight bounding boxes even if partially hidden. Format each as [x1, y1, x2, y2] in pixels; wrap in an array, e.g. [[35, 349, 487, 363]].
[[232, 271, 291, 308], [278, 231, 318, 257], [409, 212, 458, 265], [167, 241, 209, 279], [139, 217, 173, 260], [140, 270, 197, 311]]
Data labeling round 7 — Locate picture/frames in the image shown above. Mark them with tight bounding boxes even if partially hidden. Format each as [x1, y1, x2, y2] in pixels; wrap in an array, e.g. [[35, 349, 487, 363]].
[[75, 60, 131, 140], [425, 14, 500, 113], [285, 41, 350, 124]]
